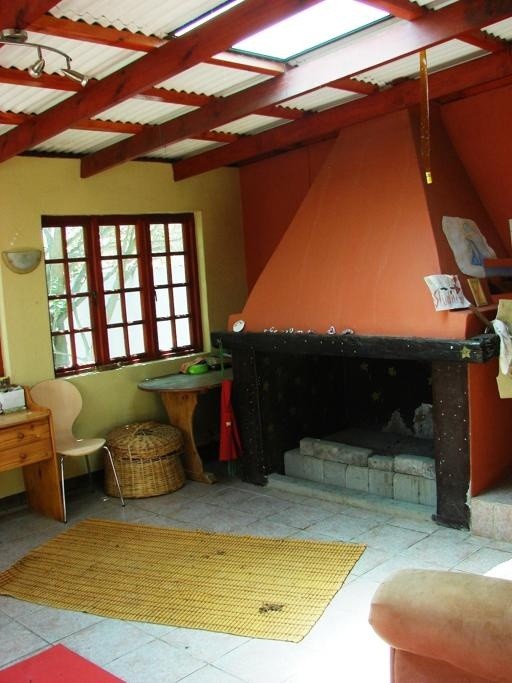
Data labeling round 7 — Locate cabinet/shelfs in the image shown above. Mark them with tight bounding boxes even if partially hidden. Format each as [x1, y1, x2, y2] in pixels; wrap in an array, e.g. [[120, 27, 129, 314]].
[[0, 382, 67, 524]]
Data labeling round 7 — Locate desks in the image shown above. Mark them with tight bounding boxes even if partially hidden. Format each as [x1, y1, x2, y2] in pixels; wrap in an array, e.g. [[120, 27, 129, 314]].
[[139, 367, 233, 484]]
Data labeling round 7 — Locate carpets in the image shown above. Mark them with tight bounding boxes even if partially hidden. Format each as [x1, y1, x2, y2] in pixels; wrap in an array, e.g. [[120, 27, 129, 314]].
[[1, 519, 369, 683]]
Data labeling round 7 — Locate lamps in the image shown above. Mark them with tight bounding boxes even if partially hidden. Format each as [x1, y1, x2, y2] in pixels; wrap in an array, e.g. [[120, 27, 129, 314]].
[[0, 28, 89, 87], [4, 249, 43, 275]]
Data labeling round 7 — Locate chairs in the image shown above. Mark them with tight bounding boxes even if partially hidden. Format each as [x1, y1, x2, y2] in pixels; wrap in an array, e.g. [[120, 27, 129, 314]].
[[27, 379, 128, 523], [367, 556, 512, 683]]
